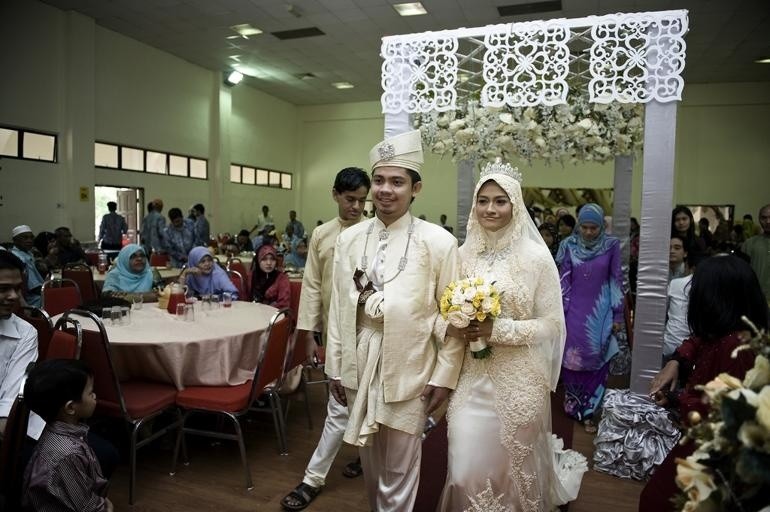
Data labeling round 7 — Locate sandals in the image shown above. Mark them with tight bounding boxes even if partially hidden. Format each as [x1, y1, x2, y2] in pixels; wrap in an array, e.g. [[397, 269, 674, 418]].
[[280, 483, 322, 510], [343, 456, 363, 477]]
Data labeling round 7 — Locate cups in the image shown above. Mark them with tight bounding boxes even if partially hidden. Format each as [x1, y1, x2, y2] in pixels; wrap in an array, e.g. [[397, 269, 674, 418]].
[[223, 291, 232, 307], [176, 303, 185, 320], [132, 296, 143, 311], [184, 305, 195, 324], [120, 306, 132, 325], [111, 305, 120, 310], [201, 295, 212, 310], [112, 310, 123, 325], [211, 294, 220, 310], [101, 307, 111, 322]]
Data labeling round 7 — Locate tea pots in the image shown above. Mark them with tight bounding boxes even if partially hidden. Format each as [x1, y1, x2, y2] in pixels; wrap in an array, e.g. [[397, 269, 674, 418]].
[[166, 284, 190, 315], [157, 282, 174, 299]]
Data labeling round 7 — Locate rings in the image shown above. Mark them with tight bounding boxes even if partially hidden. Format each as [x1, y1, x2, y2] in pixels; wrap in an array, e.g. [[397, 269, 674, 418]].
[[463, 334, 466, 339]]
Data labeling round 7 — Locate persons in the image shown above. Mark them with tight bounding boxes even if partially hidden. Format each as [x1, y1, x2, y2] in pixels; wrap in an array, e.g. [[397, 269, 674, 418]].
[[10, 225, 44, 315], [140, 202, 153, 259], [220, 230, 252, 253], [145, 198, 168, 253], [553, 202, 623, 432], [638, 253, 770, 511], [740, 204, 770, 298], [431, 157, 588, 512], [671, 204, 694, 238], [54, 227, 91, 265], [666, 236, 684, 316], [101, 243, 159, 303], [323, 134, 467, 511], [660, 240, 710, 360], [245, 245, 291, 311], [555, 214, 576, 241], [537, 223, 560, 258], [533, 206, 541, 227], [248, 205, 273, 234], [0, 250, 39, 439], [743, 215, 761, 240], [277, 166, 372, 510], [177, 246, 239, 302], [437, 214, 450, 227], [699, 218, 712, 245], [97, 202, 128, 265], [254, 224, 275, 252], [283, 236, 306, 269], [163, 208, 193, 269], [264, 235, 289, 269], [31, 231, 59, 278], [193, 204, 210, 247], [557, 209, 568, 217], [543, 208, 551, 217], [718, 224, 750, 263], [285, 211, 304, 240], [630, 216, 640, 309], [21, 356, 114, 512]]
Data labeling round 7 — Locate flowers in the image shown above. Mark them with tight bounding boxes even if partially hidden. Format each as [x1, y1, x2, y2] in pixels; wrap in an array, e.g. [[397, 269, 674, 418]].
[[438, 275, 504, 361]]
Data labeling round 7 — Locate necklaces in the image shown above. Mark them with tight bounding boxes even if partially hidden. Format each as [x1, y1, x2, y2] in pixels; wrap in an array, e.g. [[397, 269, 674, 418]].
[[361, 207, 415, 287]]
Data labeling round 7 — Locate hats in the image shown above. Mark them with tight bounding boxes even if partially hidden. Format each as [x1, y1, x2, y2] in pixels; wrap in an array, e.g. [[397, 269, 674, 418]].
[[369, 129, 424, 181], [12, 225, 32, 237]]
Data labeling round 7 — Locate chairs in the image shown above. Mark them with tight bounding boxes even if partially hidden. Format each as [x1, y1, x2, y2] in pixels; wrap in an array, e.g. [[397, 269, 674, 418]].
[[276, 329, 314, 430], [39, 277, 83, 320], [226, 257, 248, 284], [62, 308, 181, 506], [169, 306, 294, 490], [228, 269, 247, 300], [61, 263, 114, 306], [43, 315, 81, 361], [29, 305, 48, 326]]
